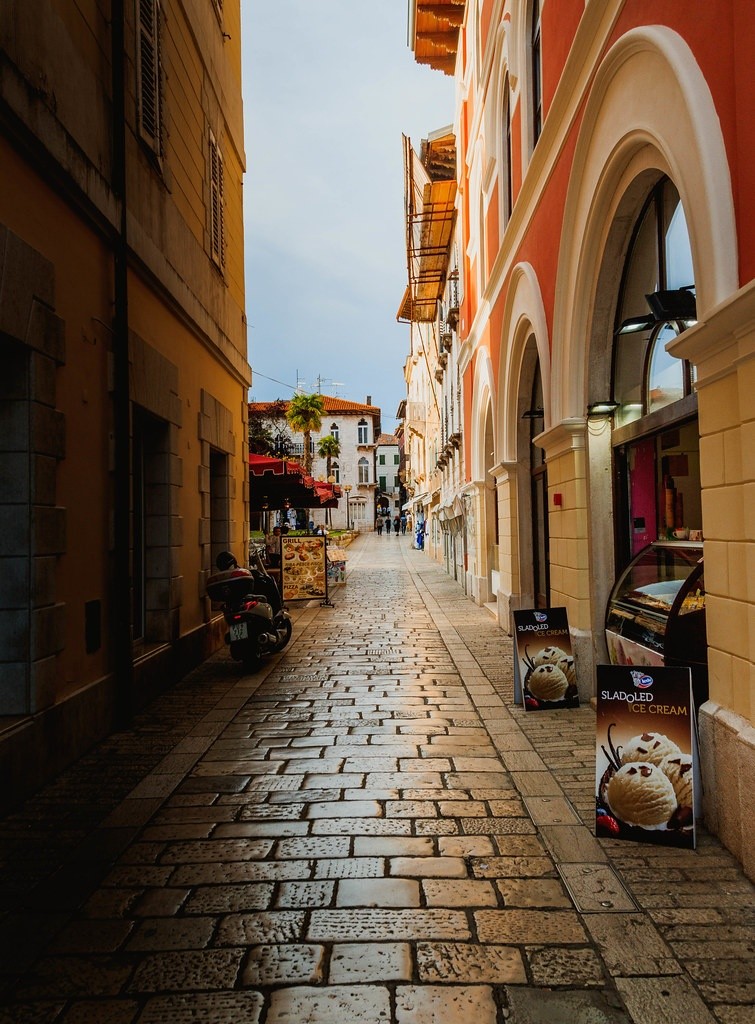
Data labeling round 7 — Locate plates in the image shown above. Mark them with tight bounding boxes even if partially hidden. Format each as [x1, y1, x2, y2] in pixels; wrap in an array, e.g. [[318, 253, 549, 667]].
[[284, 552, 325, 599]]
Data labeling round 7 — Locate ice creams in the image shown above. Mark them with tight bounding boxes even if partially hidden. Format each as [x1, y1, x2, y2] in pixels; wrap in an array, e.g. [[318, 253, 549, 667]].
[[601, 722, 693, 832], [522, 643, 577, 703]]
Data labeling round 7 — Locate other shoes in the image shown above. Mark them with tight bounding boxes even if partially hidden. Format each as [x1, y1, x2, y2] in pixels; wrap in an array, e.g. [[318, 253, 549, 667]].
[[396, 534, 399, 536]]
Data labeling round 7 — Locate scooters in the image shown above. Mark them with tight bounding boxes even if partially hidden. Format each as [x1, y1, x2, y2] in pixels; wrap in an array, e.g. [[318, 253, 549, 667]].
[[205, 542, 293, 672]]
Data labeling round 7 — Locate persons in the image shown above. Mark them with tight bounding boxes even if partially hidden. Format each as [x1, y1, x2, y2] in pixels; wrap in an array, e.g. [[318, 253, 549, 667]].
[[313, 525, 330, 535], [375, 515, 407, 536], [261, 525, 289, 565]]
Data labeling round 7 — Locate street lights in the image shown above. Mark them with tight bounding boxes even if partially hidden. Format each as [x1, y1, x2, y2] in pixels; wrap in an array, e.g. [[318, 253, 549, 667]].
[[343, 485, 352, 530]]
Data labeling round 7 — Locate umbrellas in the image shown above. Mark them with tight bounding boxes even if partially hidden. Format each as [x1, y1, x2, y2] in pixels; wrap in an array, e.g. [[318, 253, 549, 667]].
[[248, 453, 345, 509]]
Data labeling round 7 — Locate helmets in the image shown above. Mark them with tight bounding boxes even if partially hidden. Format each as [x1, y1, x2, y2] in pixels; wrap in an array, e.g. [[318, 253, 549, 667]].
[[215, 551, 238, 571]]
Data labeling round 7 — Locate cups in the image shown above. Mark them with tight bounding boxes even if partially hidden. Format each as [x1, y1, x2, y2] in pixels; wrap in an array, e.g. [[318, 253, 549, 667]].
[[673, 531, 686, 539]]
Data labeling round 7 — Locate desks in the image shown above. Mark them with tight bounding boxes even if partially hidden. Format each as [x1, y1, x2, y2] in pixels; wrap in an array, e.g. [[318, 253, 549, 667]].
[[266, 568, 280, 582]]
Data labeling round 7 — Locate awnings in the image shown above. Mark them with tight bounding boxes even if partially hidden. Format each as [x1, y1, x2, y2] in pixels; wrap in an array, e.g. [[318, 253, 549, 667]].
[[422, 487, 441, 506], [402, 494, 427, 510]]
